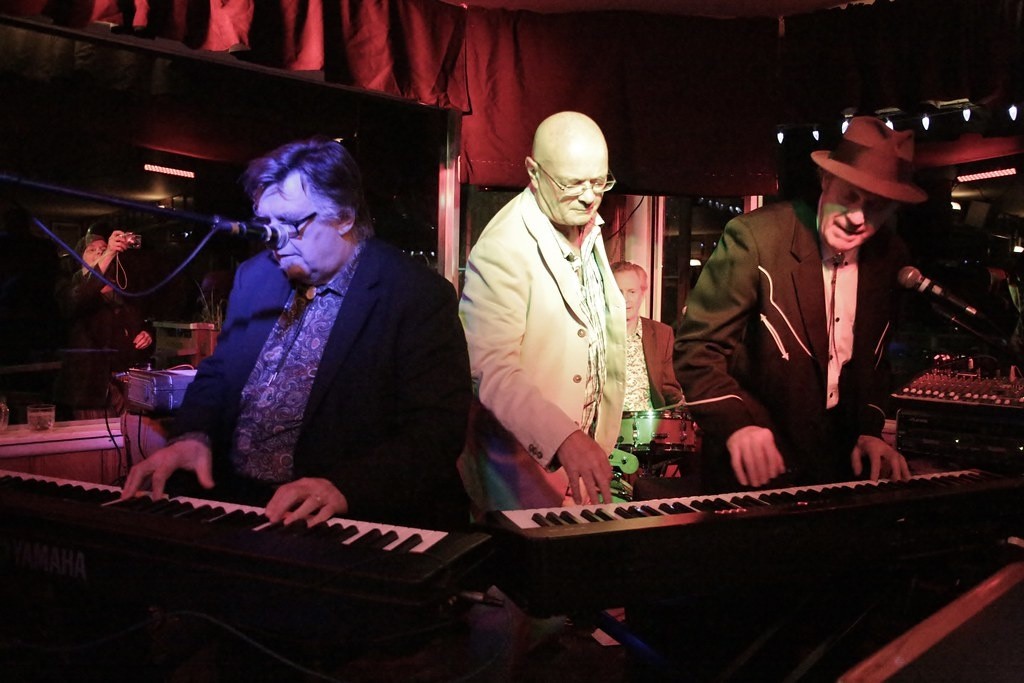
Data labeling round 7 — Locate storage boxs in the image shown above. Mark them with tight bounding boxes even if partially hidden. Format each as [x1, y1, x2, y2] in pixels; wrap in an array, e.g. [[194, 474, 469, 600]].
[[126, 370, 198, 414]]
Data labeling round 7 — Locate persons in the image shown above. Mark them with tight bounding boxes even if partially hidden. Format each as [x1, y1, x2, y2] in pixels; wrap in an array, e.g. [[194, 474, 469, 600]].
[[162, 271, 229, 337], [457, 109, 686, 504], [121, 140, 474, 529], [672, 115, 928, 488], [52, 228, 154, 373]]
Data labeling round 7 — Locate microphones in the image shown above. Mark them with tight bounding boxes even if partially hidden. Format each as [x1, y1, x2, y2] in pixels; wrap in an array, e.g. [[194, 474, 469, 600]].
[[219, 221, 289, 250], [898, 267, 982, 319]]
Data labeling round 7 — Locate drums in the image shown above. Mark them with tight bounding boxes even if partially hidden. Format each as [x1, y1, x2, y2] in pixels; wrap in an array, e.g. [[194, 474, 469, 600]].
[[617, 411, 704, 455], [568, 477, 635, 503]]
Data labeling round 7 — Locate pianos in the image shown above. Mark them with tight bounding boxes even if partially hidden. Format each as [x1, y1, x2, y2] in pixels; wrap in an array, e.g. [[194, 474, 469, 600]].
[[480, 466, 1012, 616], [0, 468, 491, 646]]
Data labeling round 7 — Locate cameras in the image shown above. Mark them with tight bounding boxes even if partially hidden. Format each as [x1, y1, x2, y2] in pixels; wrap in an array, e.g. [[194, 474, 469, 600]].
[[119, 232, 142, 249]]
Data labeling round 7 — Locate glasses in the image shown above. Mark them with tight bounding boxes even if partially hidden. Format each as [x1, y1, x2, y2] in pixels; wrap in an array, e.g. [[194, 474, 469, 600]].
[[536, 161, 616, 196], [254, 212, 317, 237]]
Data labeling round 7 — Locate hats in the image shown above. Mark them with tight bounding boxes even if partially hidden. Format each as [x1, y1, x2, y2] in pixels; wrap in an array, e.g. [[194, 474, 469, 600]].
[[810, 116, 929, 203]]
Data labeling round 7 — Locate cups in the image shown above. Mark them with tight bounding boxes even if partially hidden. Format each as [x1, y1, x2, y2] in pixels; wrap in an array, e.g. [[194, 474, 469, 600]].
[[0, 397, 9, 434], [27, 404, 56, 433]]
